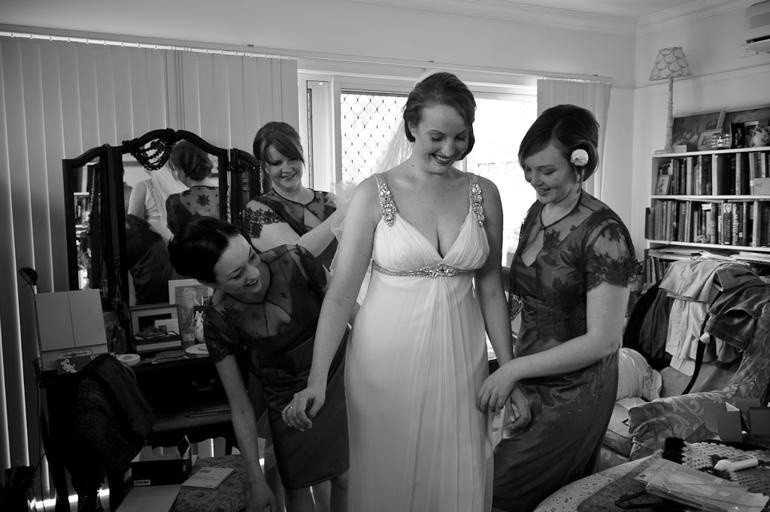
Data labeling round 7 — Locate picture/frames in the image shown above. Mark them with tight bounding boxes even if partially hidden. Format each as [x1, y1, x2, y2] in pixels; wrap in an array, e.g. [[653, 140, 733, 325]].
[[720, 102, 770, 149], [130, 304, 182, 337], [672, 110, 724, 151], [167, 279, 213, 340]]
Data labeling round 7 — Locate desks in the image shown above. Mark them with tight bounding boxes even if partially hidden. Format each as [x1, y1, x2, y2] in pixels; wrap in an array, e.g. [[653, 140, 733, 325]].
[[32, 336, 237, 512], [533, 449, 661, 512]]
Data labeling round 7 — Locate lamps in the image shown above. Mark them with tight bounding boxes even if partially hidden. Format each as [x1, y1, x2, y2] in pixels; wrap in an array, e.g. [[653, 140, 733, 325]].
[[650, 46, 692, 154], [19, 267, 41, 357]]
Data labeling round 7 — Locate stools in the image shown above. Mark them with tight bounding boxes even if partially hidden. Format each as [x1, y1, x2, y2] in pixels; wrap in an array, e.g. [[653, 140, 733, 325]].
[[171, 455, 249, 511]]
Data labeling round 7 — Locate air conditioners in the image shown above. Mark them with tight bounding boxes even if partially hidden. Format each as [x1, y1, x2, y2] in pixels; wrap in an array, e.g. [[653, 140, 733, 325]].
[[742, 0, 770, 54]]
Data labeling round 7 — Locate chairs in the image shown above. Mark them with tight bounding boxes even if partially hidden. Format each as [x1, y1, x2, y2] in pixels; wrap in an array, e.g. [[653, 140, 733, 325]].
[[595, 302, 770, 471], [88, 353, 239, 458]]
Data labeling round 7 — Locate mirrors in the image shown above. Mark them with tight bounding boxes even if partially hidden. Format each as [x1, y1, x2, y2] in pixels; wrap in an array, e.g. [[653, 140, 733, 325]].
[[63, 127, 267, 355]]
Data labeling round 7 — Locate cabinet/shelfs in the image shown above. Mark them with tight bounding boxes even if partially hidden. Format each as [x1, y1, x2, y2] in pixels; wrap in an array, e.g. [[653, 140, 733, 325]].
[[642, 146, 770, 285], [73, 161, 99, 231]]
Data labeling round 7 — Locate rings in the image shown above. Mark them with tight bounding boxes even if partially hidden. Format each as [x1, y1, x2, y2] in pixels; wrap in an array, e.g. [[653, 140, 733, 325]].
[[287, 404, 296, 413]]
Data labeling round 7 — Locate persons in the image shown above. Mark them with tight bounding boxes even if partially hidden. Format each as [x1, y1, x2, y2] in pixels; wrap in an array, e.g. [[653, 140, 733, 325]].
[[124, 141, 219, 303], [475, 103, 637, 512], [244, 121, 361, 511], [169, 214, 361, 512], [282, 71, 531, 512]]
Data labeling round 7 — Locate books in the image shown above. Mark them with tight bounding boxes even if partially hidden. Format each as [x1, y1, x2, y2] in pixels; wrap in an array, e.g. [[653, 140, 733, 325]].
[[645, 152, 769, 249]]
